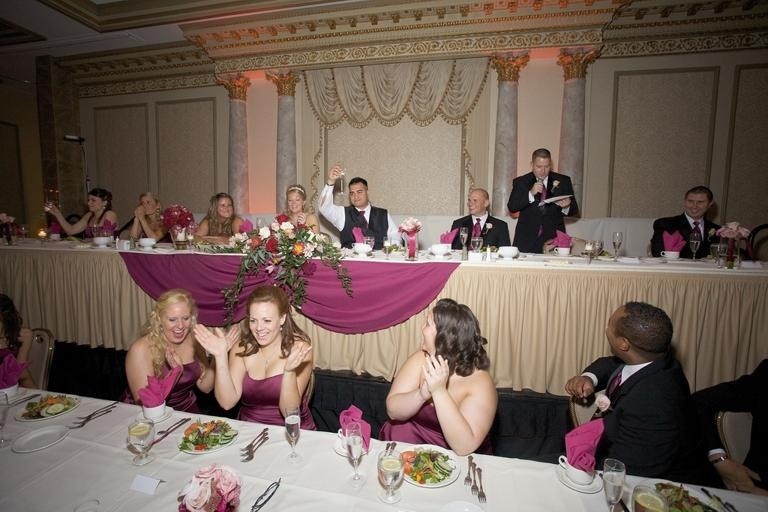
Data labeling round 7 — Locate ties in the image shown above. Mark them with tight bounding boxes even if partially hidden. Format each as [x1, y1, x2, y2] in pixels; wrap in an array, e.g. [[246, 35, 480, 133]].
[[359, 211, 367, 228], [605, 371, 622, 397], [540, 184, 548, 203], [692, 221, 702, 241], [471, 218, 482, 237]]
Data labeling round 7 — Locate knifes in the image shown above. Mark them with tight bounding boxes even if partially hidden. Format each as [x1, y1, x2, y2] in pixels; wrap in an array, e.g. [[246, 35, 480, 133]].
[[154, 417, 192, 444], [240, 432, 268, 457]]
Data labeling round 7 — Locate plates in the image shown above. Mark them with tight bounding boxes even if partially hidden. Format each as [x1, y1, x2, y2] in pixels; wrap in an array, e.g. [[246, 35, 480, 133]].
[[134, 406, 174, 425], [399, 443, 462, 489], [0, 385, 28, 405], [331, 439, 376, 457], [10, 424, 71, 455], [640, 477, 725, 512], [175, 427, 241, 455], [13, 393, 83, 422], [554, 467, 606, 494], [666, 257, 685, 262]]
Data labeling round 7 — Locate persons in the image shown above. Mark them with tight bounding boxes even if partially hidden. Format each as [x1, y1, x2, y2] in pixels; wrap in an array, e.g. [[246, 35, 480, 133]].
[[282, 186, 318, 233], [380, 297, 497, 456], [506, 150, 580, 252], [194, 193, 245, 243], [687, 358, 768, 498], [43, 188, 119, 240], [650, 187, 721, 259], [128, 191, 172, 243], [451, 189, 510, 251], [191, 286, 316, 430], [320, 163, 396, 251], [125, 288, 240, 416], [0, 296, 37, 389], [566, 301, 691, 482]]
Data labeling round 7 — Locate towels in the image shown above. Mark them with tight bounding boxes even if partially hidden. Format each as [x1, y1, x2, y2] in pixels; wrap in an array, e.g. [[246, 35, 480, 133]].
[[351, 226, 365, 243], [440, 227, 460, 244], [338, 404, 372, 454], [552, 230, 573, 248], [102, 218, 118, 234], [662, 230, 686, 252], [49, 222, 61, 234], [137, 365, 181, 407], [564, 417, 606, 473], [0, 352, 34, 389], [238, 218, 254, 233]]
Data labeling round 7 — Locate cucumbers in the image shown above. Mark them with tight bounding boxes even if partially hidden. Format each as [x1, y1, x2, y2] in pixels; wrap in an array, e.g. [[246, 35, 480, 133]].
[[41, 403, 66, 417], [218, 430, 238, 444], [432, 460, 451, 480]]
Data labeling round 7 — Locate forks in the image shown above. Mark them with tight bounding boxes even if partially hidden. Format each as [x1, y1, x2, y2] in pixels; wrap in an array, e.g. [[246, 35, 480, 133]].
[[470, 462, 479, 496], [71, 404, 117, 424], [73, 401, 120, 420], [463, 455, 474, 487], [240, 427, 268, 452], [66, 410, 113, 430], [476, 466, 488, 505]]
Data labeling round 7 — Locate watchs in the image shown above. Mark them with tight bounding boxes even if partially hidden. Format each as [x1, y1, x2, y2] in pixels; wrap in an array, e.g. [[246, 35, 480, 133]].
[[327, 183, 335, 186]]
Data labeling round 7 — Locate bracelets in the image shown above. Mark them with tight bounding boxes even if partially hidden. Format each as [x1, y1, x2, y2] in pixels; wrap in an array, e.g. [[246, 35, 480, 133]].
[[710, 454, 728, 464]]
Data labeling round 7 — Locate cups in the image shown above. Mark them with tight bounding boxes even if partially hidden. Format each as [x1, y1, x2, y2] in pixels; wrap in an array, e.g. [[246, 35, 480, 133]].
[[459, 226, 469, 249], [584, 240, 593, 263], [602, 458, 626, 511], [0, 389, 12, 450], [34, 227, 50, 248], [470, 236, 484, 253], [46, 189, 56, 206], [50, 234, 60, 239], [383, 237, 393, 260], [352, 242, 373, 253], [375, 450, 406, 506], [126, 418, 157, 467], [363, 235, 375, 252], [554, 248, 570, 255], [90, 222, 100, 238], [343, 422, 367, 489], [141, 399, 167, 420], [282, 405, 303, 464], [710, 244, 718, 265], [611, 232, 623, 262], [537, 174, 545, 184], [631, 484, 670, 512], [255, 215, 266, 228], [338, 426, 345, 447], [392, 240, 400, 259], [18, 223, 30, 243], [661, 250, 680, 260], [717, 243, 728, 268], [557, 455, 596, 486], [118, 239, 130, 251], [102, 228, 112, 237], [430, 243, 451, 258], [688, 231, 701, 263], [336, 163, 349, 196], [592, 240, 604, 261]]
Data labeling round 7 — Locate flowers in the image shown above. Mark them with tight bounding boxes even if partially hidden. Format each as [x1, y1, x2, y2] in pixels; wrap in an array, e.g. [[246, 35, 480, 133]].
[[158, 203, 195, 229], [397, 216, 423, 233], [206, 213, 353, 333], [708, 220, 751, 238]]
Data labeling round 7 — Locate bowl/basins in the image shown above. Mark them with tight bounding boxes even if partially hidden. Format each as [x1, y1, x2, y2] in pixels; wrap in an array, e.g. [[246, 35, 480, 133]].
[[139, 238, 156, 249], [0, 382, 19, 398], [93, 237, 111, 248], [498, 246, 518, 260]]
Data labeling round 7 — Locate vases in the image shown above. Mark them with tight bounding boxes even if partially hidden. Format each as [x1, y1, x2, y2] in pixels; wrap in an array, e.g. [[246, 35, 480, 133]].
[[722, 239, 740, 270], [168, 229, 188, 251], [404, 233, 418, 261]]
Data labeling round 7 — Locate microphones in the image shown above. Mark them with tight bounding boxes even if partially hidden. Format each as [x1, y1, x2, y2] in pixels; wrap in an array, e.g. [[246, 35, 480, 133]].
[[538, 175, 543, 193]]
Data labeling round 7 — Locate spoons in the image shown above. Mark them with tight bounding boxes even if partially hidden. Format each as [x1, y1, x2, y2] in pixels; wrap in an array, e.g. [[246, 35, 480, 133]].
[[240, 437, 269, 462], [158, 417, 186, 435]]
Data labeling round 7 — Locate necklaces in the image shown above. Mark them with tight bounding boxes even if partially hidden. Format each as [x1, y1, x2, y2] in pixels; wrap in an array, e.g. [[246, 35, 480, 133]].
[[258, 346, 280, 365]]
